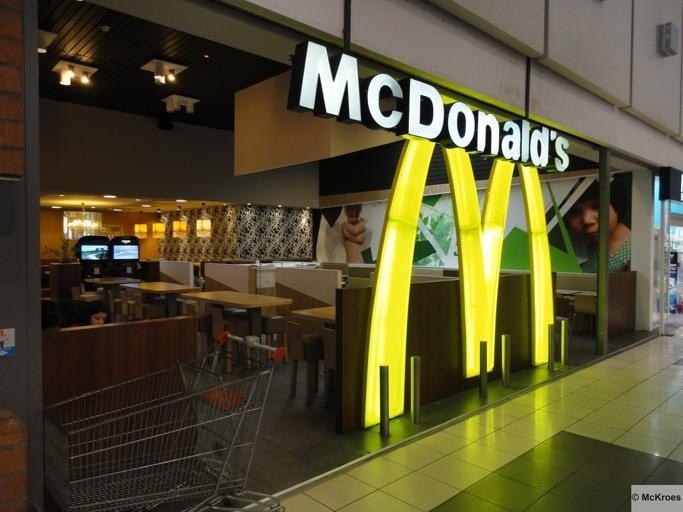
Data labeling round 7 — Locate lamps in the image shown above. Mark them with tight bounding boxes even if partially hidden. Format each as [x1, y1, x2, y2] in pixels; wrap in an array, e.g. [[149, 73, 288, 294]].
[[161, 94, 200, 114], [140, 58, 189, 85], [134, 203, 211, 238], [52, 60, 98, 85]]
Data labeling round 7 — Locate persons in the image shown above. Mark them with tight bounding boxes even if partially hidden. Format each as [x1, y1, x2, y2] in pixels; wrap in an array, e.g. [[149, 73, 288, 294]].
[[68, 300, 113, 327], [340, 204, 367, 263], [561, 176, 632, 273]]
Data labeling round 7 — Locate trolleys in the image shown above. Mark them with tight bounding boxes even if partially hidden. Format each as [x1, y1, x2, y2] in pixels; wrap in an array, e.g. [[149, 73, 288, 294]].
[[44, 331, 285, 511]]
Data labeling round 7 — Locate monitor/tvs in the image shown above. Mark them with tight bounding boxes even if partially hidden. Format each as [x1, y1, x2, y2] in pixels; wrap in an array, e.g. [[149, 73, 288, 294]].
[[81, 243, 109, 261], [113, 245, 139, 260]]
